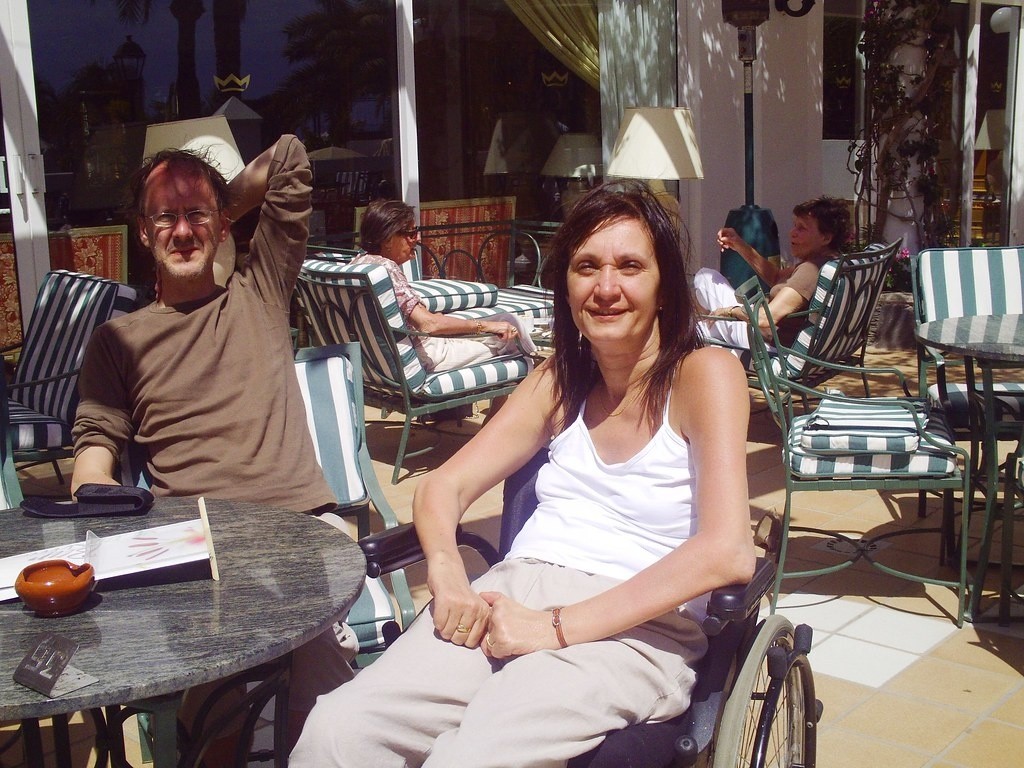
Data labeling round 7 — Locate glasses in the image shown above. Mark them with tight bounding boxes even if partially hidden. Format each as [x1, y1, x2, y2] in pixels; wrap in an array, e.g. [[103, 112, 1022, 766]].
[[141, 210, 220, 228], [396, 226, 419, 239]]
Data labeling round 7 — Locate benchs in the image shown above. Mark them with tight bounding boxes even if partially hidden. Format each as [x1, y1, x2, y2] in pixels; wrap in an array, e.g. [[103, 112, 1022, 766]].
[[292, 219, 565, 349]]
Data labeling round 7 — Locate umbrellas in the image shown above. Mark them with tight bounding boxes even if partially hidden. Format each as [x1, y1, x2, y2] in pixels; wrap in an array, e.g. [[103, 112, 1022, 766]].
[[306, 144, 368, 161]]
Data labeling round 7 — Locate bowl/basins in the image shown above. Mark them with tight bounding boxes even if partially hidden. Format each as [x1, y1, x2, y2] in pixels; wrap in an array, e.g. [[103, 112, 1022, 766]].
[[15, 559, 94, 617]]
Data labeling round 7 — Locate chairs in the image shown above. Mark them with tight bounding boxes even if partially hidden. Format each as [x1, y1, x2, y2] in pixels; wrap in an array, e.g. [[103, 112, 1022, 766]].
[[694, 237, 904, 414], [733, 277, 970, 627], [111, 340, 418, 768], [908, 245, 1024, 564], [326, 156, 391, 236], [0, 268, 139, 489], [291, 266, 533, 485]]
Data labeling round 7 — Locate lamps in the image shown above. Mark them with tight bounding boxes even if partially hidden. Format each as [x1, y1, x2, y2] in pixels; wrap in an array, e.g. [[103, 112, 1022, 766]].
[[482, 112, 557, 219], [142, 114, 247, 185], [973, 108, 1005, 202], [536, 133, 605, 222], [67, 120, 146, 222], [605, 107, 706, 248]]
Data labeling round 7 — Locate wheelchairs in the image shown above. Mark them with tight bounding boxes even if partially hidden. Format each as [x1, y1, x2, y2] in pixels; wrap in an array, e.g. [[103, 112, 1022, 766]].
[[359, 444, 824, 768]]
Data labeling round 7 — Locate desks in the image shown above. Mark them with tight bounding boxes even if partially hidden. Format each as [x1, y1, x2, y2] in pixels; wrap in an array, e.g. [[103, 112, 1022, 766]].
[[914, 313, 1024, 622], [0, 496, 368, 768]]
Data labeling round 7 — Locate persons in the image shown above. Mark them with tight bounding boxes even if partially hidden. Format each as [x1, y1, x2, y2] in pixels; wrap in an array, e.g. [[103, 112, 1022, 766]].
[[351, 200, 537, 429], [67, 135, 359, 768], [689, 198, 852, 357], [289, 180, 755, 768]]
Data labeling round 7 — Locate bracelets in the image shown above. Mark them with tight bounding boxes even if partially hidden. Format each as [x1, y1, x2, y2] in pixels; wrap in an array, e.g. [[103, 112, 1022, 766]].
[[473, 316, 483, 337], [727, 305, 739, 315], [551, 606, 568, 651]]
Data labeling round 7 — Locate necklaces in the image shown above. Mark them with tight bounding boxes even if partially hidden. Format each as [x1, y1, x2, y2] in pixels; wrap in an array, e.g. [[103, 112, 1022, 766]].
[[597, 378, 651, 416]]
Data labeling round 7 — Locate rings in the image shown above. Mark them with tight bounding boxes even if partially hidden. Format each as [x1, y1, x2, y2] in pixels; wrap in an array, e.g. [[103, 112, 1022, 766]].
[[512, 326, 516, 331], [457, 623, 471, 633], [485, 633, 492, 648]]
